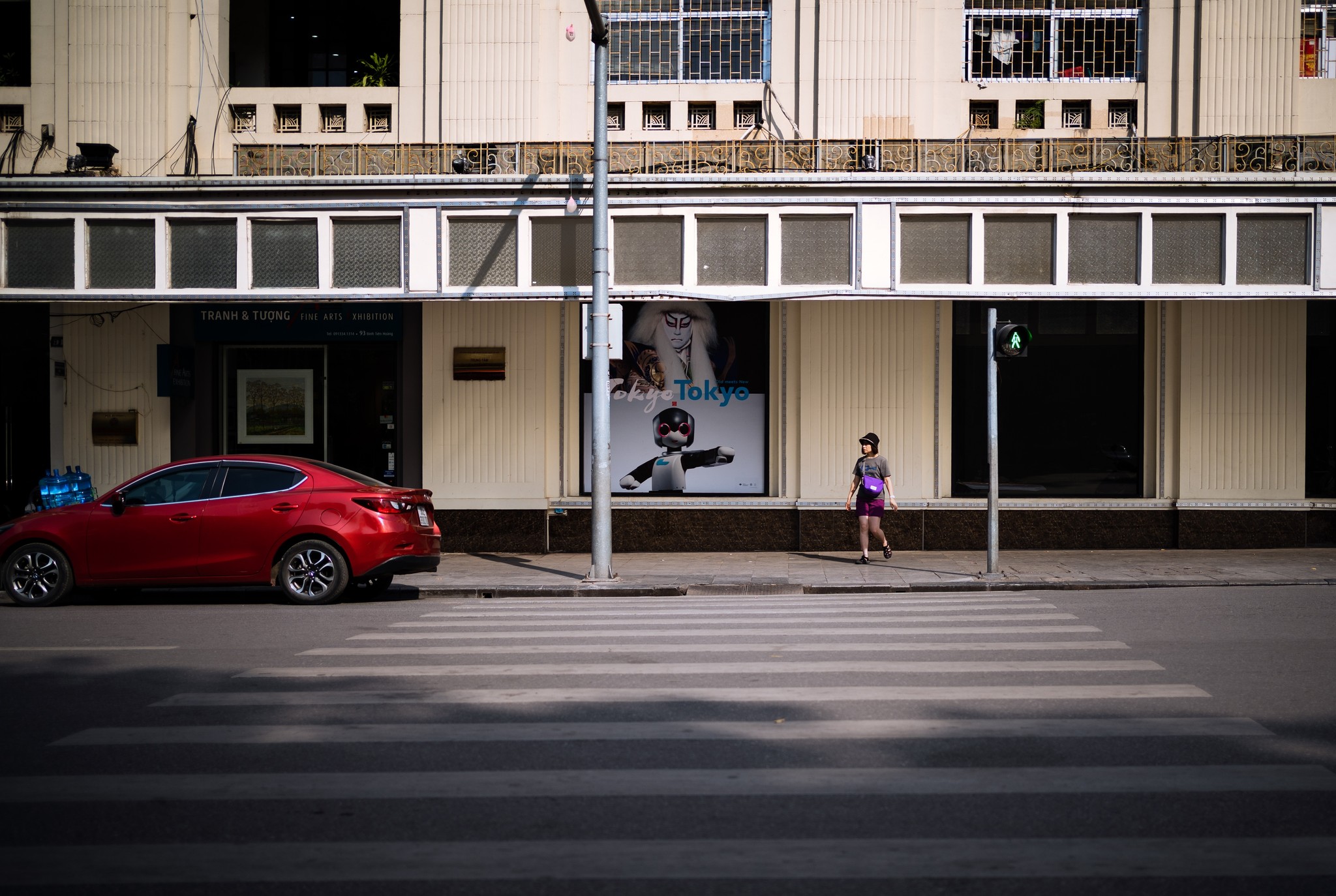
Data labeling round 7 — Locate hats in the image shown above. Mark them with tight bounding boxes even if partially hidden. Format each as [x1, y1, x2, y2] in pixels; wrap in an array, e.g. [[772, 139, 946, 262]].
[[859, 432, 880, 449]]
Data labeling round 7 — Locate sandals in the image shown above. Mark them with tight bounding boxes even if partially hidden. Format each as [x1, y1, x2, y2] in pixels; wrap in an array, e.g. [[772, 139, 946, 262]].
[[882, 540, 892, 559], [855, 555, 871, 564]]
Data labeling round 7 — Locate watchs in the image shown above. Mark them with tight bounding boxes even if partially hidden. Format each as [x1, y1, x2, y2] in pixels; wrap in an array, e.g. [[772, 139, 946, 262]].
[[889, 495, 895, 498]]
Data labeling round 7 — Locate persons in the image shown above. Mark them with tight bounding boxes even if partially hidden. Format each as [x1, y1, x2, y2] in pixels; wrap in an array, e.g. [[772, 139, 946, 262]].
[[845, 433, 897, 564]]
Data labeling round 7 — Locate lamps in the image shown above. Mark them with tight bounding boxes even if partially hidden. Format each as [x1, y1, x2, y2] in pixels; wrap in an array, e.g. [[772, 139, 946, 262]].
[[861, 154, 877, 171], [847, 138, 883, 173], [1231, 140, 1272, 170], [462, 143, 499, 175], [459, 157, 470, 175], [74, 140, 121, 171], [65, 155, 79, 173], [451, 157, 466, 175]]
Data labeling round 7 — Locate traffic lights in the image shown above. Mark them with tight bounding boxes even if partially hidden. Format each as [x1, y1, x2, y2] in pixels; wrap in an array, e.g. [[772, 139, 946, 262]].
[[994, 321, 1031, 360]]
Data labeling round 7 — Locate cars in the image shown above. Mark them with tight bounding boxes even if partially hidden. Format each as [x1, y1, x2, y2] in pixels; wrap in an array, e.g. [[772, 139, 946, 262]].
[[0, 455, 441, 606]]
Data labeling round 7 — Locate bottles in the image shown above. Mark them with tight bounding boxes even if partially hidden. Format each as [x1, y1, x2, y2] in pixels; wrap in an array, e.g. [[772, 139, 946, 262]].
[[47, 469, 73, 508], [62, 466, 76, 483], [46, 506, 50, 509], [39, 469, 54, 506], [80, 498, 85, 503], [37, 506, 41, 511], [69, 466, 94, 504]]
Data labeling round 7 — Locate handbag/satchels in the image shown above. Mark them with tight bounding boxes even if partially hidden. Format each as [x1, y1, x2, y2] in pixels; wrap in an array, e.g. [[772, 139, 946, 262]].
[[861, 475, 884, 494]]
